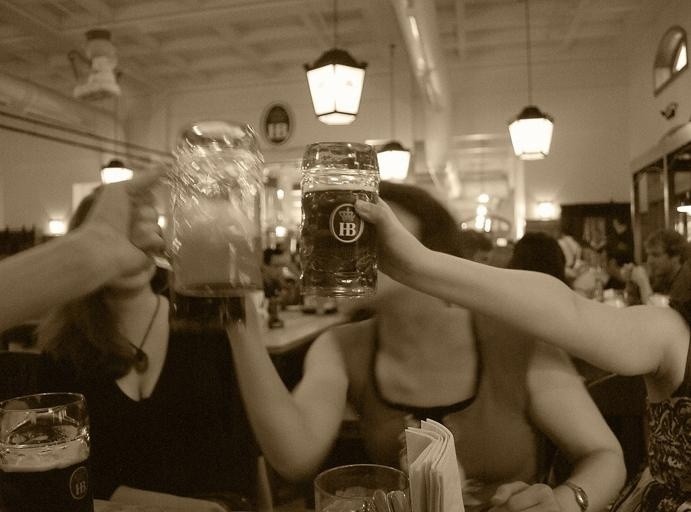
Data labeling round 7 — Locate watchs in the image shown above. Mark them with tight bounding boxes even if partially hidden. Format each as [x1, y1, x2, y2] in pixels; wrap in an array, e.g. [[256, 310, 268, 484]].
[[562, 480, 589, 512]]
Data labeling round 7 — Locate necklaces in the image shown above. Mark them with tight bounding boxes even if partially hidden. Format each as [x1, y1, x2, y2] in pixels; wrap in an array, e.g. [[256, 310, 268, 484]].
[[119, 294, 162, 374]]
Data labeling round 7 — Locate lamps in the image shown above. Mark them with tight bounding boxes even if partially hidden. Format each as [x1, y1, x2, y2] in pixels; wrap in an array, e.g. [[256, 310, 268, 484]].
[[99, 63, 137, 189], [377, 42, 413, 183], [304, 0, 369, 125], [508, 3, 555, 161]]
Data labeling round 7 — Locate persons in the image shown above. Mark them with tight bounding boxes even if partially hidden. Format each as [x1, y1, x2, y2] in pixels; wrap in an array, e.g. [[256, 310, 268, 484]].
[[34, 183, 276, 511], [355, 193, 691, 510], [262, 248, 285, 297], [218, 179, 628, 511], [457, 227, 688, 321], [0, 163, 169, 331]]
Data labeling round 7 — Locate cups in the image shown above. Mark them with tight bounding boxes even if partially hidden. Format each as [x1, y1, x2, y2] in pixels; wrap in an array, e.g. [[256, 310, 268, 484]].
[[123, 116, 266, 301], [313, 464, 412, 511], [1, 389, 95, 512], [296, 139, 383, 302]]
